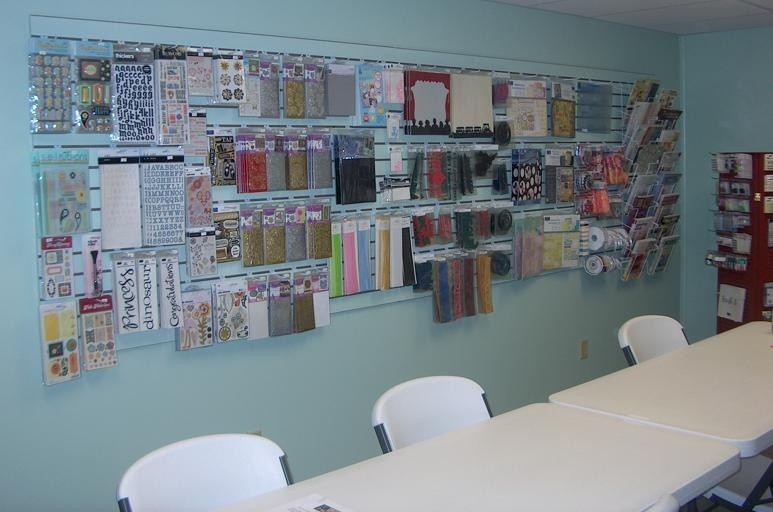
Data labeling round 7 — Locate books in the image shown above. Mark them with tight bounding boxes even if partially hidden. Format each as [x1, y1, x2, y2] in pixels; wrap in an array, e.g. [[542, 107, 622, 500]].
[[621, 76, 683, 281]]
